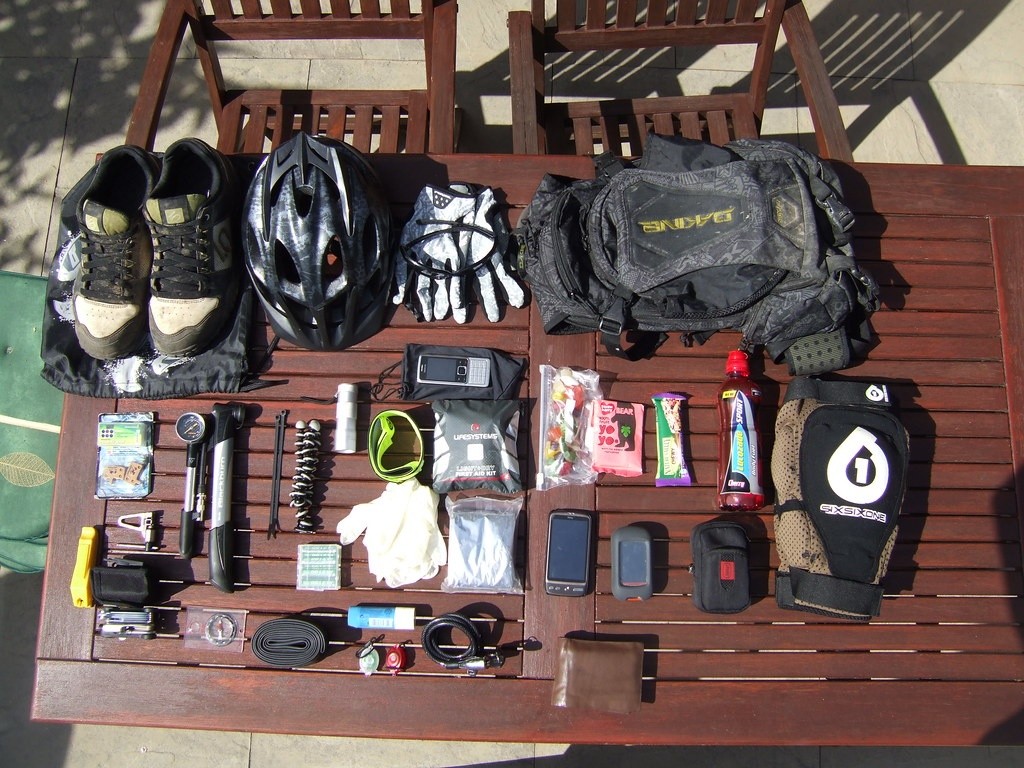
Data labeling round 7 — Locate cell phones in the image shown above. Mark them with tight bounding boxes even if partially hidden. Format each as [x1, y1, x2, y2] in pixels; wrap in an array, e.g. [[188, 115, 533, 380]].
[[416, 355, 490, 387], [612, 527, 653, 601], [545, 510, 593, 596]]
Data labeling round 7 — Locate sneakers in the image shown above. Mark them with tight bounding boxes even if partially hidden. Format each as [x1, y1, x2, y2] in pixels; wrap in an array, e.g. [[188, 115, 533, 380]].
[[71, 138, 240, 359]]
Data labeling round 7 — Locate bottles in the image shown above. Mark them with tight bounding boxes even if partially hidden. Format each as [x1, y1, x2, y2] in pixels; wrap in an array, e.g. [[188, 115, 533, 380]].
[[715, 349, 765, 510]]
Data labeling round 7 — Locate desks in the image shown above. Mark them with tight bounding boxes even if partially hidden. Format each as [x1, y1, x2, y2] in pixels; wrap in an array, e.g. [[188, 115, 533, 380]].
[[29, 153, 1024, 747]]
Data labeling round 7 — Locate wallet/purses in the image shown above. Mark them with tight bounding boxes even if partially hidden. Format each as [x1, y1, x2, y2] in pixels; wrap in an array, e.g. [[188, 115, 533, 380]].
[[554, 636, 645, 715]]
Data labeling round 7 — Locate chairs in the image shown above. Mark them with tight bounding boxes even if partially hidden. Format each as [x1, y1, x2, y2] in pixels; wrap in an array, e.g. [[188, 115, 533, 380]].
[[124, 0, 459, 154], [508, 0, 856, 163]]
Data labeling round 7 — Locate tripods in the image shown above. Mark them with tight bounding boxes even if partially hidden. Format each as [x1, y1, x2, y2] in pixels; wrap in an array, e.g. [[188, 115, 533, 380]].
[[290, 419, 323, 532]]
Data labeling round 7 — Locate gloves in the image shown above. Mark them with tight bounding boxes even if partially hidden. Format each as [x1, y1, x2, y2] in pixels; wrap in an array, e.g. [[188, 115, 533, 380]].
[[392, 179, 525, 326]]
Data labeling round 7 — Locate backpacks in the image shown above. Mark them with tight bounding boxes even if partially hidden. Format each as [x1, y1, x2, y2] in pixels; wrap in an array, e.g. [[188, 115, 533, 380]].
[[512, 128, 882, 376]]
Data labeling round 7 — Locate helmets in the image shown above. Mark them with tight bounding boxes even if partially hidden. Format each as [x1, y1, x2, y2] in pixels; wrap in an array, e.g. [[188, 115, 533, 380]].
[[240, 130, 398, 352]]
